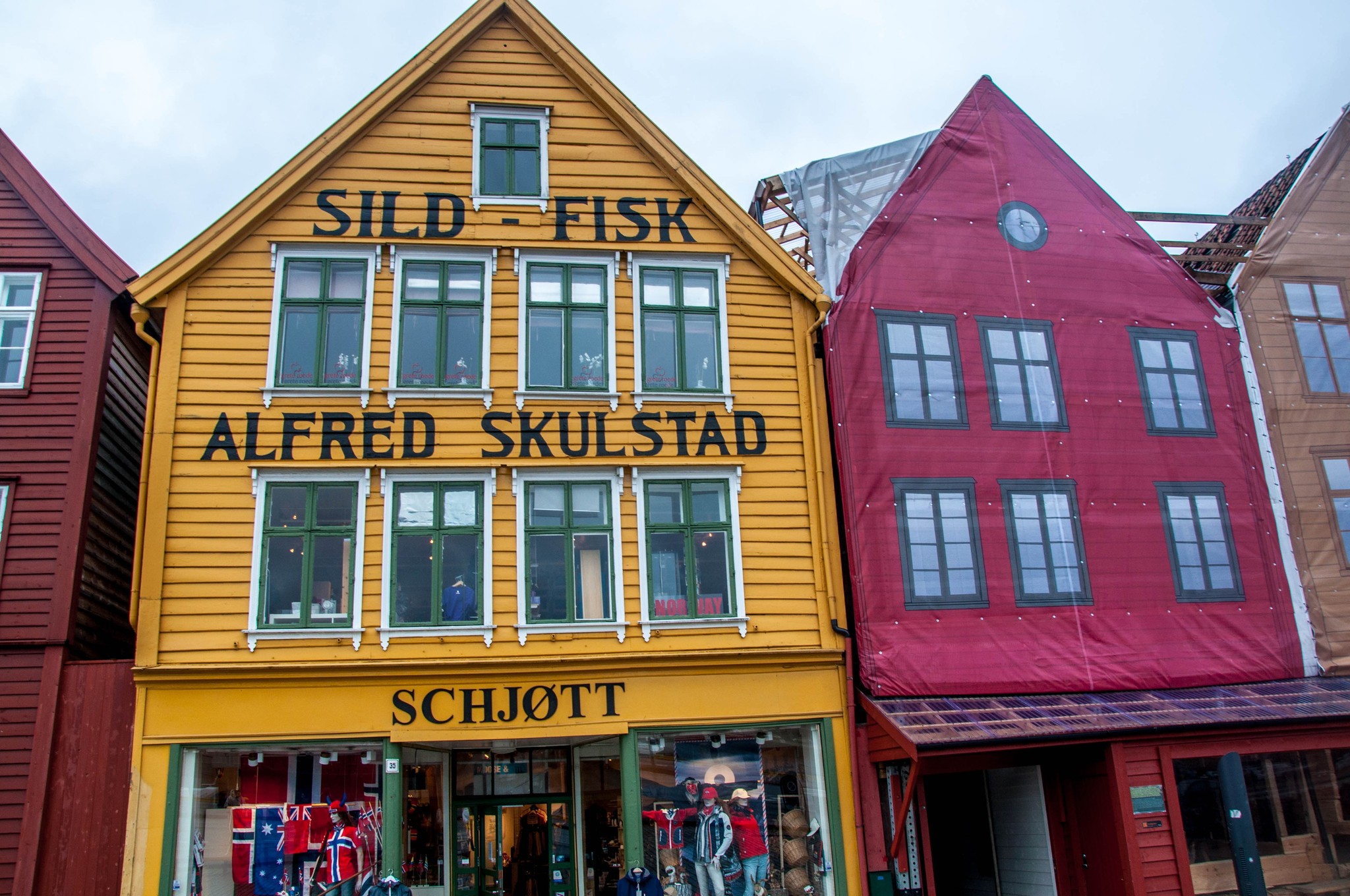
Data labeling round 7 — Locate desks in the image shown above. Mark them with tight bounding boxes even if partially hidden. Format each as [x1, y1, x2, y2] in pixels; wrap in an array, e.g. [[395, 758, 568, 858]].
[[268, 614, 348, 624]]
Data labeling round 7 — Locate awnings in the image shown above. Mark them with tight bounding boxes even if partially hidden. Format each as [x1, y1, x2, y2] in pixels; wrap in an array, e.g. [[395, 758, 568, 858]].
[[860, 677, 1350, 860]]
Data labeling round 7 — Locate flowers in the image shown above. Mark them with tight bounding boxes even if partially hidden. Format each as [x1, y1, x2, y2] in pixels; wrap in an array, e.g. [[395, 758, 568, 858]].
[[502, 852, 512, 868]]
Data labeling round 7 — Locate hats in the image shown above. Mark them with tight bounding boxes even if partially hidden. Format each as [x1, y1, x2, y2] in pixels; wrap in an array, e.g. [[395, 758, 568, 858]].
[[732, 789, 752, 800], [701, 787, 718, 799], [327, 794, 347, 813]]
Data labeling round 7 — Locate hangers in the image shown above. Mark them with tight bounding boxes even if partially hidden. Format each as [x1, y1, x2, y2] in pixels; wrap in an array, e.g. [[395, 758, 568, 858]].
[[453, 575, 466, 587], [384, 869, 397, 883], [632, 860, 643, 873], [528, 804, 538, 814]]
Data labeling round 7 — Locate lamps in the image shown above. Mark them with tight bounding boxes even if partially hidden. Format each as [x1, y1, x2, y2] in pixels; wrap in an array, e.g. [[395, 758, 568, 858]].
[[319, 752, 338, 765], [711, 734, 726, 748], [248, 752, 264, 767], [649, 737, 666, 751], [491, 740, 516, 754], [361, 751, 376, 764], [756, 731, 773, 744]]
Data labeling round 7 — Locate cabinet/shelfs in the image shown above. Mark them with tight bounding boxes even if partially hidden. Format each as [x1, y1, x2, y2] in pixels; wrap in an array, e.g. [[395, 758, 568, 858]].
[[610, 825, 619, 868]]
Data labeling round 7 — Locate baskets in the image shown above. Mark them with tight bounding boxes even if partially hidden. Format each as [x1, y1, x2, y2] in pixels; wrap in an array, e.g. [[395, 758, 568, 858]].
[[657, 874, 681, 888], [784, 868, 811, 896], [777, 809, 809, 839], [660, 848, 680, 874], [782, 839, 809, 868]]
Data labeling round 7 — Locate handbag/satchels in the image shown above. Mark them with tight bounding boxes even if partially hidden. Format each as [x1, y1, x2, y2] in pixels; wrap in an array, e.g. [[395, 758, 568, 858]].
[[720, 850, 743, 883]]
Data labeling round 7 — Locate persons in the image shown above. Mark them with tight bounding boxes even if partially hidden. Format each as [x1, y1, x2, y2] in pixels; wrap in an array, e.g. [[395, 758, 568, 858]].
[[692, 787, 733, 896], [729, 789, 768, 896], [309, 794, 363, 896]]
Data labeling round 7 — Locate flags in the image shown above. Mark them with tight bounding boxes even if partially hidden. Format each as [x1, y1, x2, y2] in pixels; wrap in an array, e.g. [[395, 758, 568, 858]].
[[231, 801, 381, 896]]
